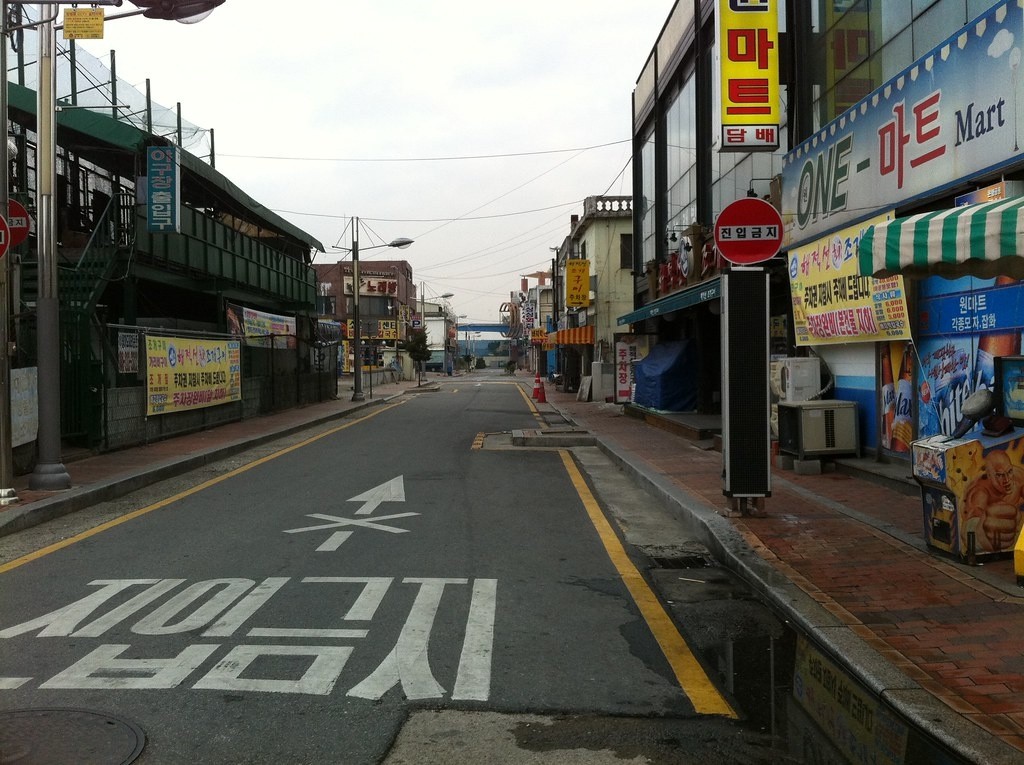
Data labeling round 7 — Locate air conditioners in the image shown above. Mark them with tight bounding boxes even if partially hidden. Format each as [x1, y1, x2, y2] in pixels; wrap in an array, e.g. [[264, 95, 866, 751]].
[[779, 358, 822, 401], [779, 399, 859, 459]]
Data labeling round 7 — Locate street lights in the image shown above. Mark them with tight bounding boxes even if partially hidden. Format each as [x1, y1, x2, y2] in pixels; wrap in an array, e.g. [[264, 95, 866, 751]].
[[29, 0, 226, 494], [331, 217, 416, 402], [454, 313, 482, 374], [409, 280, 455, 382]]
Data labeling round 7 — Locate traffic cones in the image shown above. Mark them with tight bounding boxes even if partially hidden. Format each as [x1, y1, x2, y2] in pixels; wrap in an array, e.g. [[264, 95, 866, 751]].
[[531, 372, 540, 399], [536, 381, 549, 404]]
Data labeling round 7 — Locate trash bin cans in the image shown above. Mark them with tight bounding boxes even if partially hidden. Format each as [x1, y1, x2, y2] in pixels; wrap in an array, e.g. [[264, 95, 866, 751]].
[[447, 366, 452, 375]]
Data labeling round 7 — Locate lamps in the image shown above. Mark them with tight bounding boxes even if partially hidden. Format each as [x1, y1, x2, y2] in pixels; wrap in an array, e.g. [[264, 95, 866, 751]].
[[664, 225, 691, 251], [747, 177, 774, 197], [641, 269, 650, 278]]
